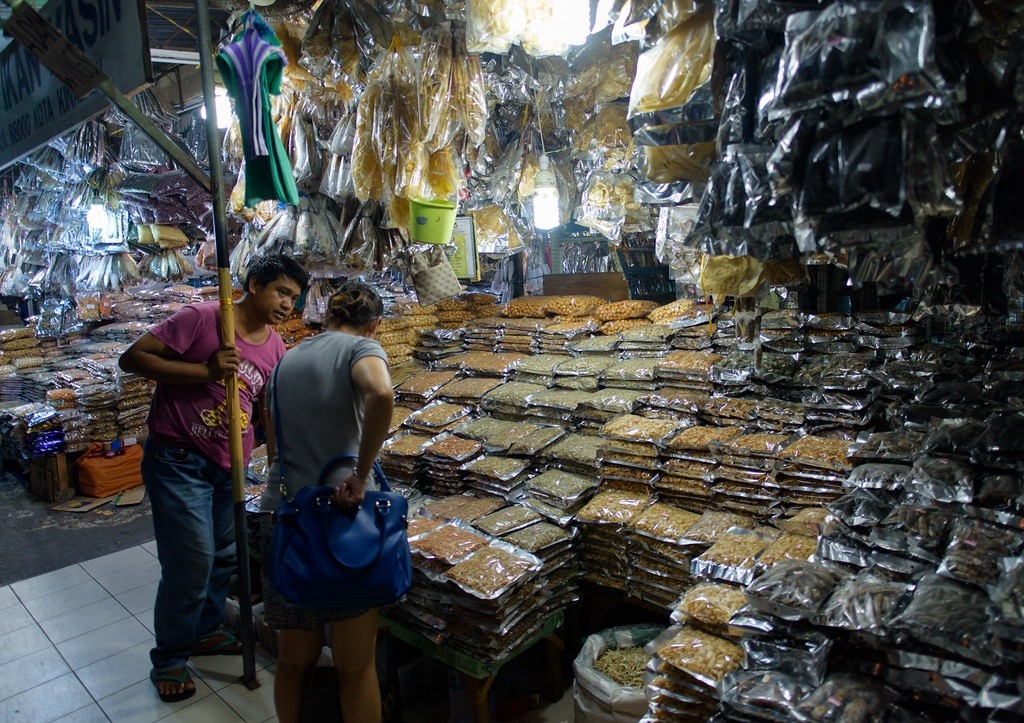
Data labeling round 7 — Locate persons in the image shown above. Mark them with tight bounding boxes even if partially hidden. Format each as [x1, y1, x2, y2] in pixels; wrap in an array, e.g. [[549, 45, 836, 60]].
[[118, 253, 308, 700], [264, 281, 395, 722]]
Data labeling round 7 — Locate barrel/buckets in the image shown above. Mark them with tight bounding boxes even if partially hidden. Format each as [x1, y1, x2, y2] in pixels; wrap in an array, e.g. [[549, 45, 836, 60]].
[[408, 170, 459, 245]]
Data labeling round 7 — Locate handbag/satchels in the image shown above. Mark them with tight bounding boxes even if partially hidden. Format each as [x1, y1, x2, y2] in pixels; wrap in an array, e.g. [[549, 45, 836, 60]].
[[268, 452, 414, 611], [410, 246, 463, 308]]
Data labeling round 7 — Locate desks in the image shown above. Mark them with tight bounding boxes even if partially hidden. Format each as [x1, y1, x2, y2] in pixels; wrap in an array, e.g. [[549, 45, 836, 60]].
[[31, 449, 86, 505], [381, 607, 567, 723]]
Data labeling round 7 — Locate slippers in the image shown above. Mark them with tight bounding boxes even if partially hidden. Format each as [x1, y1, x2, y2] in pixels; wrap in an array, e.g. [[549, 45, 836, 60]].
[[150, 666, 196, 702], [193, 632, 242, 655]]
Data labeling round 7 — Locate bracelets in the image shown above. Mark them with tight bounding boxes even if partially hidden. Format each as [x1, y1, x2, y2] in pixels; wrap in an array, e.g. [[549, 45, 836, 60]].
[[351, 466, 373, 487]]
